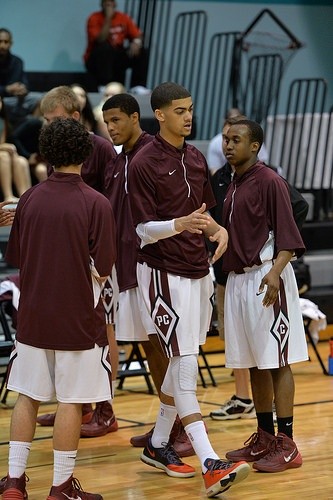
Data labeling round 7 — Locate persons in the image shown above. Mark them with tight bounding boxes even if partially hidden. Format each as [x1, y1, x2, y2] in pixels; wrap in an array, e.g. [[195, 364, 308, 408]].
[[102, 80, 252, 498], [0, 85, 119, 500], [212, 120, 307, 474], [0, 0, 283, 208]]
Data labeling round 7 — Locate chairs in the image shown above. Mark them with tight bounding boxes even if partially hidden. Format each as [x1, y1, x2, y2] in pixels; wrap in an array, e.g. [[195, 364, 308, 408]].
[[0, 276, 327, 404]]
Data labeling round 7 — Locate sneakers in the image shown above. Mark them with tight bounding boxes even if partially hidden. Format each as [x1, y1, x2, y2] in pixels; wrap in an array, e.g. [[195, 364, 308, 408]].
[[253, 432, 303, 472], [36, 403, 93, 425], [98, 86, 106, 95], [210, 394, 258, 421], [172, 421, 208, 456], [140, 434, 196, 478], [47, 474, 103, 500], [0, 471, 29, 500], [1, 198, 20, 211], [80, 401, 118, 436], [130, 414, 180, 447], [130, 85, 152, 96], [226, 427, 277, 462], [272, 403, 279, 427], [201, 458, 250, 498]]
